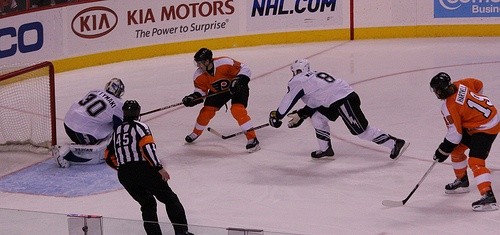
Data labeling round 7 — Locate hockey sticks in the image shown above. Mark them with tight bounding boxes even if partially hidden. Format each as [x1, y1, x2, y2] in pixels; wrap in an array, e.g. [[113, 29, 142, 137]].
[[207, 122, 270, 140], [139, 88, 230, 117], [381, 158, 439, 208]]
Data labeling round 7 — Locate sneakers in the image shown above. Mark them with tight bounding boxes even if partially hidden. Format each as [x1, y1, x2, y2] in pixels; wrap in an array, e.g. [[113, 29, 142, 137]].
[[471, 190, 498, 212], [185, 132, 200, 143], [246, 138, 261, 152], [310, 150, 335, 160], [52, 146, 68, 168], [444, 177, 471, 195], [389, 137, 409, 160]]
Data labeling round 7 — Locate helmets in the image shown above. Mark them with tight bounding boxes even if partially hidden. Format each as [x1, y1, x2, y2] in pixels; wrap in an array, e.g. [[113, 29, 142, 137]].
[[104, 77, 124, 98], [291, 59, 310, 76], [122, 100, 141, 119], [194, 47, 213, 62], [430, 72, 457, 99]]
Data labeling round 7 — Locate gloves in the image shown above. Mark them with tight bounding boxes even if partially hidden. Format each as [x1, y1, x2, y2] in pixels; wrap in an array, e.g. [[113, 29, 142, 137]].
[[234, 75, 251, 88], [287, 110, 304, 128], [181, 92, 204, 107], [431, 143, 451, 163], [268, 110, 283, 129]]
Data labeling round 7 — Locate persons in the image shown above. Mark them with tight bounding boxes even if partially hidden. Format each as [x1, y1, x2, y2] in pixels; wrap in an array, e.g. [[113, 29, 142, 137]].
[[104, 99, 194, 235], [269, 58, 410, 160], [429, 72, 500, 212], [182, 48, 261, 153], [52, 77, 126, 167]]
